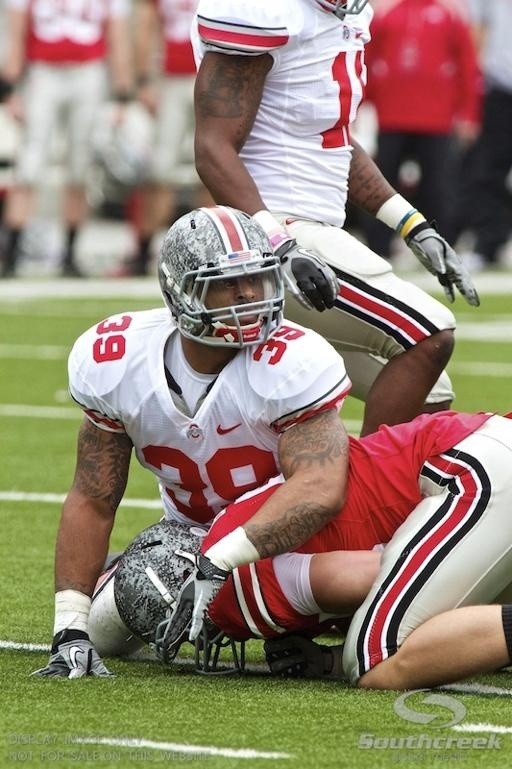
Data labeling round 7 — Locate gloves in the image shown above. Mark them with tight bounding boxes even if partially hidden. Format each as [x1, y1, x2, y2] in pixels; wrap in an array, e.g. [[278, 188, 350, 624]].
[[160, 549, 231, 665], [264, 632, 333, 679], [273, 237, 341, 313], [394, 209, 479, 307], [29, 627, 115, 679]]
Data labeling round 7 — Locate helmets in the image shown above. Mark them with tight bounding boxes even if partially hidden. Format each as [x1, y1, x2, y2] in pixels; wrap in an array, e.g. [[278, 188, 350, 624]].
[[111, 521, 229, 661], [154, 205, 286, 350]]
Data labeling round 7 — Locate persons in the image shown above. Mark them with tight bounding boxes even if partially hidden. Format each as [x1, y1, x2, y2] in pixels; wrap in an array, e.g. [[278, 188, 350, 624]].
[[0, 0, 512, 276], [187, 0, 480, 439], [27, 205, 353, 683], [112, 410, 511, 691]]
